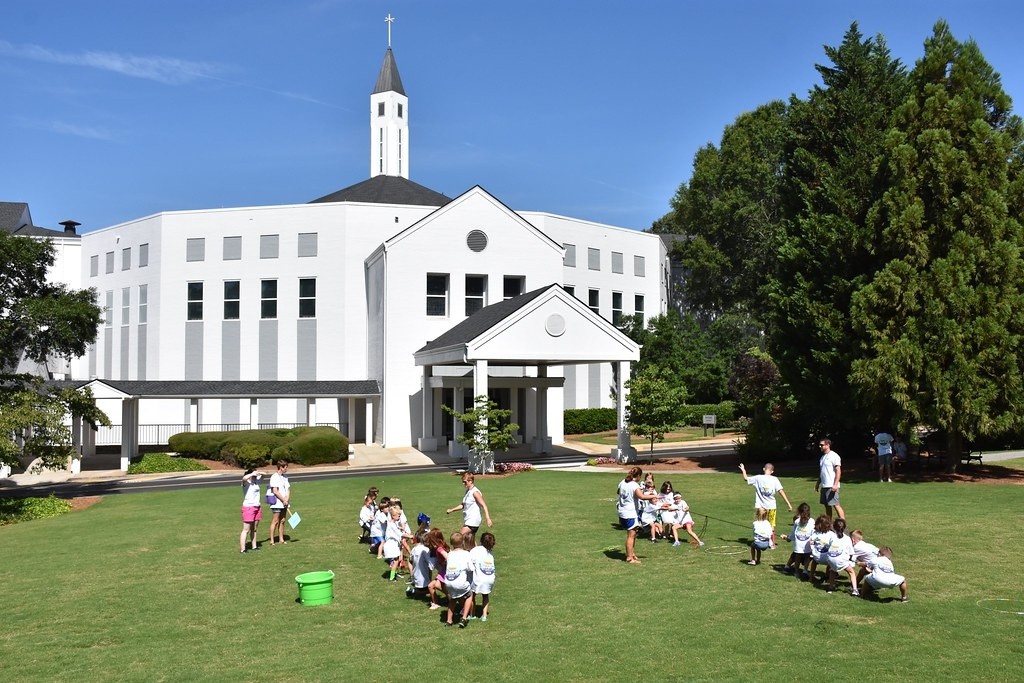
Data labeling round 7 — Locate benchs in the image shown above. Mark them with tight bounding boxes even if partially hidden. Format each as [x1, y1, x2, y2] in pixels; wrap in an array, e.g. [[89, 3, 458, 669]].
[[919, 444, 982, 466]]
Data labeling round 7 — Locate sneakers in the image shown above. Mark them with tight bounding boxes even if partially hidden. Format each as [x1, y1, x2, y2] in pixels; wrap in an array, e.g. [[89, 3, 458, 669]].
[[467, 616, 477, 620], [479, 616, 488, 622], [458, 618, 468, 629], [445, 621, 454, 627]]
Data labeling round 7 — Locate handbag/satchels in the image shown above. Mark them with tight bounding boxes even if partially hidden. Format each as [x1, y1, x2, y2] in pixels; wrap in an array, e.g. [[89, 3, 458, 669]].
[[265, 487, 277, 504]]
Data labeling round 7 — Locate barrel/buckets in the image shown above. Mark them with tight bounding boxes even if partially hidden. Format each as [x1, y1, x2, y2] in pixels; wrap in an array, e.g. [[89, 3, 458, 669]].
[[294, 570, 335, 607]]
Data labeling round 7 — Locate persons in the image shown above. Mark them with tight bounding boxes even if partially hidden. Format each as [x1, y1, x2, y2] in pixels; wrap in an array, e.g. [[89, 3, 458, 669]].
[[747, 508, 772, 565], [616, 467, 666, 563], [638, 473, 674, 543], [668, 491, 704, 546], [738, 463, 793, 549], [875, 426, 907, 482], [240, 468, 266, 554], [445, 532, 473, 627], [780, 501, 910, 603], [269, 459, 290, 546], [446, 471, 493, 547], [814, 438, 845, 519], [358, 487, 412, 582], [467, 533, 496, 620], [405, 513, 477, 615]]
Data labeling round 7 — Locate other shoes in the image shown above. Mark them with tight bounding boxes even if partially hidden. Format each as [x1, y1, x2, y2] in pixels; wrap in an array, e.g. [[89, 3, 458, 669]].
[[626, 558, 641, 564], [901, 598, 910, 603], [784, 562, 867, 598], [240, 550, 249, 555], [672, 542, 681, 547], [756, 561, 761, 565], [888, 479, 893, 483], [358, 535, 464, 616], [698, 542, 705, 547], [281, 541, 287, 545], [270, 543, 275, 546], [252, 548, 261, 551], [748, 560, 756, 566], [632, 555, 638, 560], [880, 479, 884, 482], [651, 533, 675, 543]]
